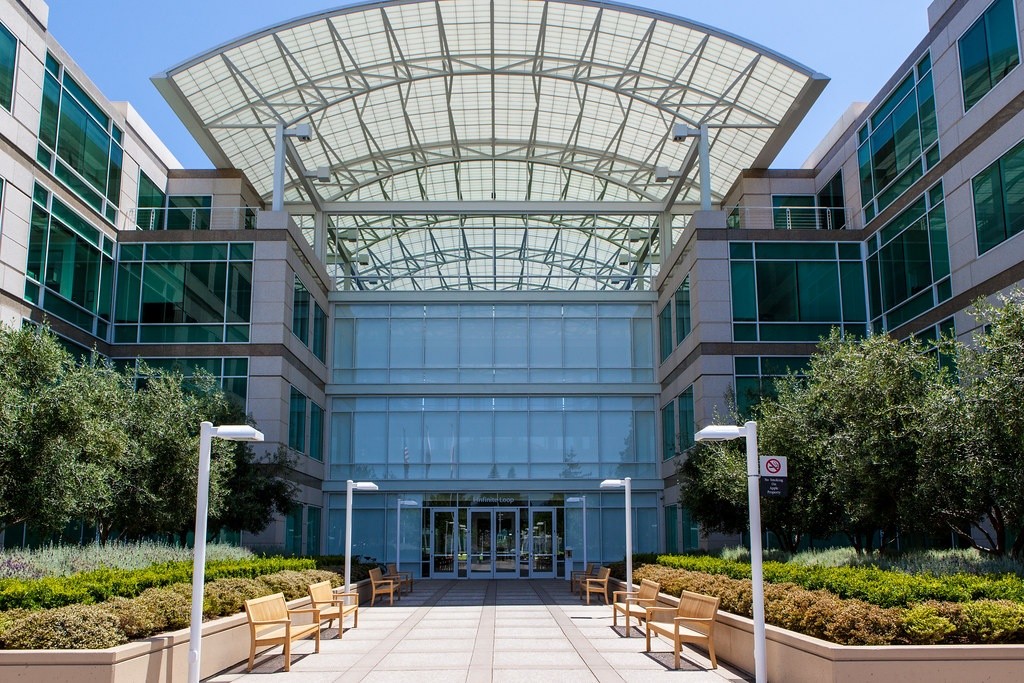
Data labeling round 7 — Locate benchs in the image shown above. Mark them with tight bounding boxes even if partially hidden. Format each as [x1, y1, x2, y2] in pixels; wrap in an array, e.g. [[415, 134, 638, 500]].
[[570, 563, 594, 595], [369, 567, 401, 607], [579, 566, 611, 606], [244, 592, 321, 671], [613, 578, 661, 638], [388, 564, 413, 596], [308, 580, 359, 639], [645, 589, 720, 671]]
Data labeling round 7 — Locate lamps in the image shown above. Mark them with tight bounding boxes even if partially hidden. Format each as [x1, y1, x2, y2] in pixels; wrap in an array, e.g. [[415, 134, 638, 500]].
[[296, 123, 312, 142], [347, 231, 379, 285], [671, 123, 701, 142], [611, 230, 639, 284], [317, 167, 331, 182], [655, 166, 681, 182]]
